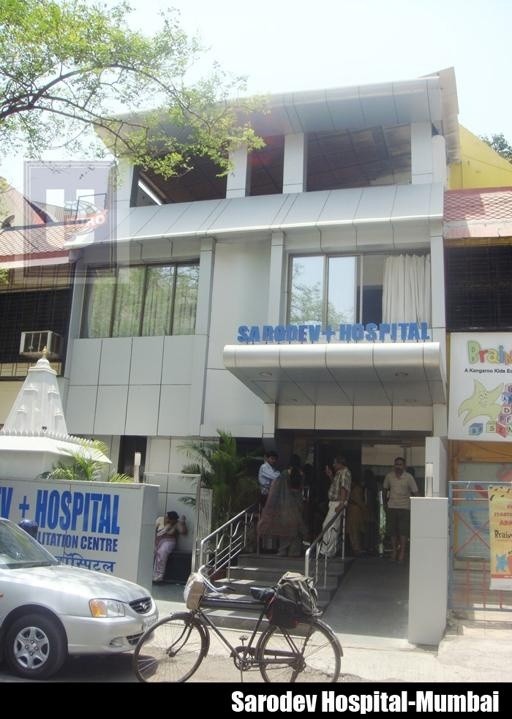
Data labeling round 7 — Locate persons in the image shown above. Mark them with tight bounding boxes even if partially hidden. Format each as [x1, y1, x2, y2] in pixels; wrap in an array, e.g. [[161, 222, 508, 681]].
[[319, 455, 353, 555], [264, 454, 311, 555], [153, 510, 188, 584], [382, 456, 420, 565], [299, 462, 379, 557], [257, 451, 280, 510]]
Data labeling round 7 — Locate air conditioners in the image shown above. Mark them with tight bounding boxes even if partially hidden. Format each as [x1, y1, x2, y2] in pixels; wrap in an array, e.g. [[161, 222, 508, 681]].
[[16, 330, 63, 359]]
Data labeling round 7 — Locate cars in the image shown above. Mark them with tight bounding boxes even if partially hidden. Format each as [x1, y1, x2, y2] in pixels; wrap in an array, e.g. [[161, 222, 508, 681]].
[[0, 516, 158, 679]]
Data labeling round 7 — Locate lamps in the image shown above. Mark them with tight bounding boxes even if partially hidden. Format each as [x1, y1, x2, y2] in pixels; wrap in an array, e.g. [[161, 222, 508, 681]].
[[133, 173, 166, 208]]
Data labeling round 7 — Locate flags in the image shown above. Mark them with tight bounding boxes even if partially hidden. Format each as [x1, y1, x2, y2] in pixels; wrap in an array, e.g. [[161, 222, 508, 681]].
[[487, 484, 512, 591]]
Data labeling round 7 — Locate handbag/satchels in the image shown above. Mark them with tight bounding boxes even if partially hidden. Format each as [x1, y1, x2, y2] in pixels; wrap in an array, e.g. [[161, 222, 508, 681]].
[[268, 570, 318, 628]]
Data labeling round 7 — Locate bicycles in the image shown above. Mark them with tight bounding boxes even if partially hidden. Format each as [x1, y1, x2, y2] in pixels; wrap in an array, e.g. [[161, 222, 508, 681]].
[[129, 562, 343, 683]]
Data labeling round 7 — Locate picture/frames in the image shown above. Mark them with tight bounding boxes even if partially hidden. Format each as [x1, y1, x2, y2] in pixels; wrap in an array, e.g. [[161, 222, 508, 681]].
[[5, 154, 136, 296]]
